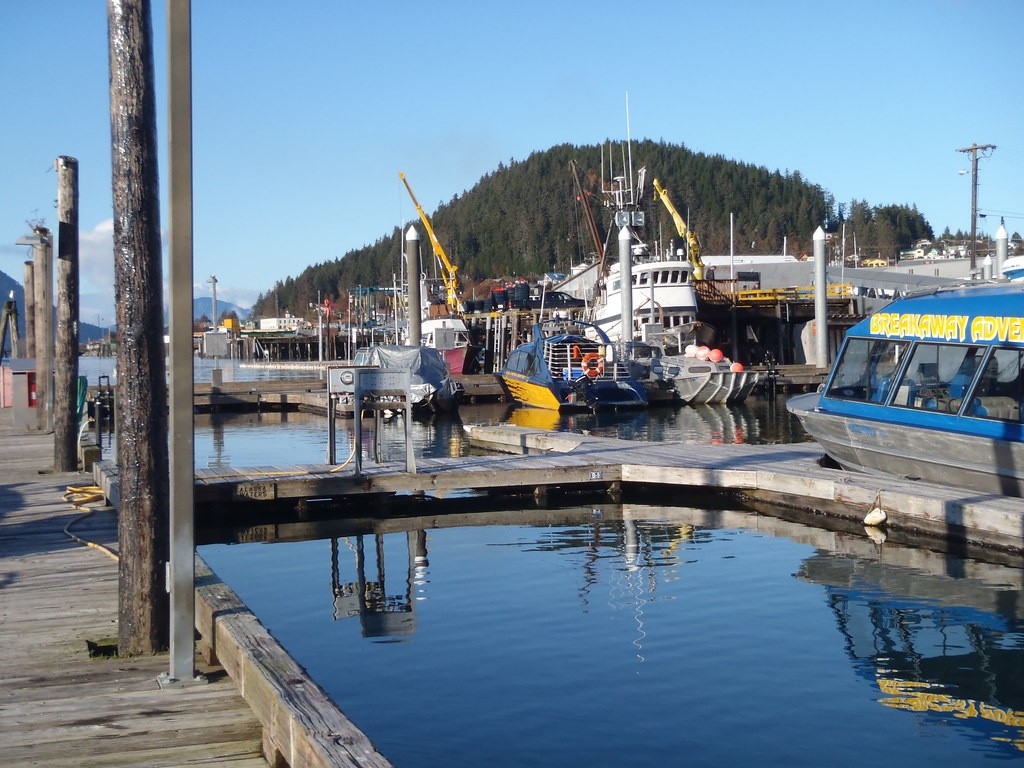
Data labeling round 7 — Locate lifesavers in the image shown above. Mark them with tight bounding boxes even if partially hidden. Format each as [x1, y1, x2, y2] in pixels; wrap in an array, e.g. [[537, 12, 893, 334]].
[[581, 352, 605, 377]]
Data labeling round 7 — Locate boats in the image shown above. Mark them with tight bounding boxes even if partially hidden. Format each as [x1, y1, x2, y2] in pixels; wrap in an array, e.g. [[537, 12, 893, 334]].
[[785, 280, 1024, 501], [790, 543, 1024, 765], [583, 89, 760, 406], [495, 318, 652, 417]]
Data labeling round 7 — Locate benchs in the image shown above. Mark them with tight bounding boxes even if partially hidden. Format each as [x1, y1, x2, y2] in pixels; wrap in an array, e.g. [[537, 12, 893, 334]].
[[949, 396, 1019, 421]]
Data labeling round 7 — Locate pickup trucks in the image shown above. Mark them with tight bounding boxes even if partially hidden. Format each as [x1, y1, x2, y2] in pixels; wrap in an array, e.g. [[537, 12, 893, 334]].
[[520, 291, 593, 311]]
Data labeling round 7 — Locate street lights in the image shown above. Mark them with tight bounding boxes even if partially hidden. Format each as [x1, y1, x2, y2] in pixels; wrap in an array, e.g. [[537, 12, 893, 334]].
[[207, 274, 223, 387], [958, 169, 980, 280]]
